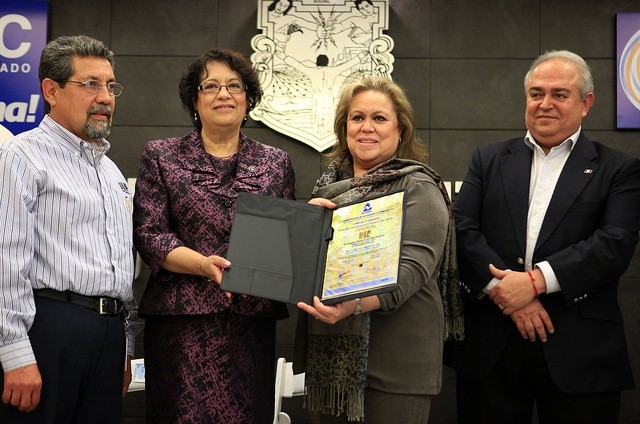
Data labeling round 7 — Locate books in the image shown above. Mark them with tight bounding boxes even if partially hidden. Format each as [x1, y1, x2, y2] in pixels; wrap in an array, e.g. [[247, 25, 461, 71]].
[[220, 188, 407, 305]]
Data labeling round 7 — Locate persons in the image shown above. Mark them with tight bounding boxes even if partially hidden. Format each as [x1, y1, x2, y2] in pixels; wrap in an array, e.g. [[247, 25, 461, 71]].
[[297, 77, 449, 423], [132, 48, 336, 424], [0, 36, 133, 424], [456, 50, 635, 424]]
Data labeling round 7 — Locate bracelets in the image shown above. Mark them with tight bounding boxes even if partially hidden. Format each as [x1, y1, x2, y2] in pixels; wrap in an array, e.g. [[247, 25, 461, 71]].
[[527, 270, 539, 297], [199, 257, 213, 282]]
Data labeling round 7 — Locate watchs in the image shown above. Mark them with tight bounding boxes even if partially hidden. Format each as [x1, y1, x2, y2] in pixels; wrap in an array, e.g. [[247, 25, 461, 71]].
[[354, 298, 362, 317]]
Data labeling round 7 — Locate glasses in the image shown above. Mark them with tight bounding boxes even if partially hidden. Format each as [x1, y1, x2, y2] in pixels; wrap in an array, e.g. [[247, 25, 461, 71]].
[[56, 80, 123, 97], [198, 79, 249, 93]]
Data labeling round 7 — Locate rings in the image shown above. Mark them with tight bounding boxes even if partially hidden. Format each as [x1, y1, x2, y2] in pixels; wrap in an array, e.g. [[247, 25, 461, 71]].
[[315, 313, 320, 320], [499, 304, 504, 311]]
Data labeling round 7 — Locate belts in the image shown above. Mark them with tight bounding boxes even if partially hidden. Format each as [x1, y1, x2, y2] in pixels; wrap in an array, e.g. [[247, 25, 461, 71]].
[[34, 289, 123, 315]]
